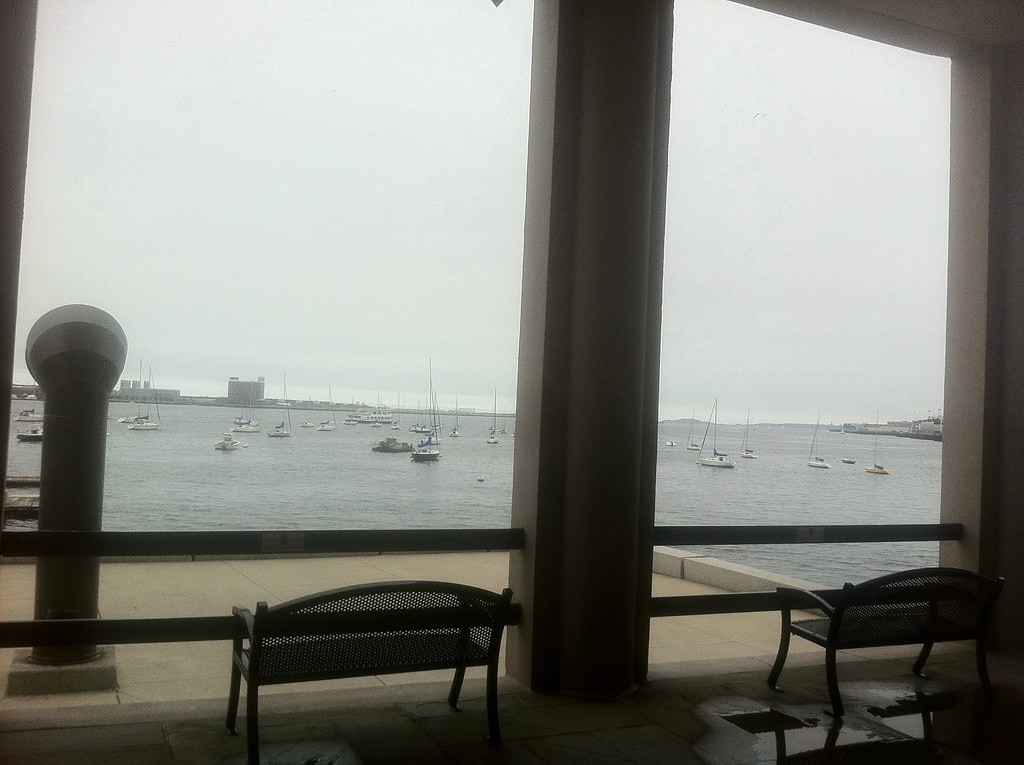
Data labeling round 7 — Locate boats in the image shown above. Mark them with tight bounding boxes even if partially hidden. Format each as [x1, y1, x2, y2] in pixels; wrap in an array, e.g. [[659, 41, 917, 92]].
[[371, 437, 415, 453], [17, 427, 43, 441], [214, 432, 240, 450]]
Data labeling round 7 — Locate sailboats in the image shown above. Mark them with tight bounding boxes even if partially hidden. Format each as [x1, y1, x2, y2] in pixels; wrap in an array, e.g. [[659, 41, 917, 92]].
[[265, 370, 294, 438], [117, 359, 162, 430], [299, 422, 315, 428], [12, 381, 44, 422], [447, 397, 461, 438], [311, 385, 337, 433], [660, 389, 897, 478], [486, 386, 508, 444], [408, 357, 446, 461], [343, 393, 403, 430], [230, 379, 261, 433]]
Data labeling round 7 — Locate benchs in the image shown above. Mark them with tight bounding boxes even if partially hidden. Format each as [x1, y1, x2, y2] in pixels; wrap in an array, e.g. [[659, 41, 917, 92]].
[[225, 581, 513, 765], [769, 567, 1005, 718]]
